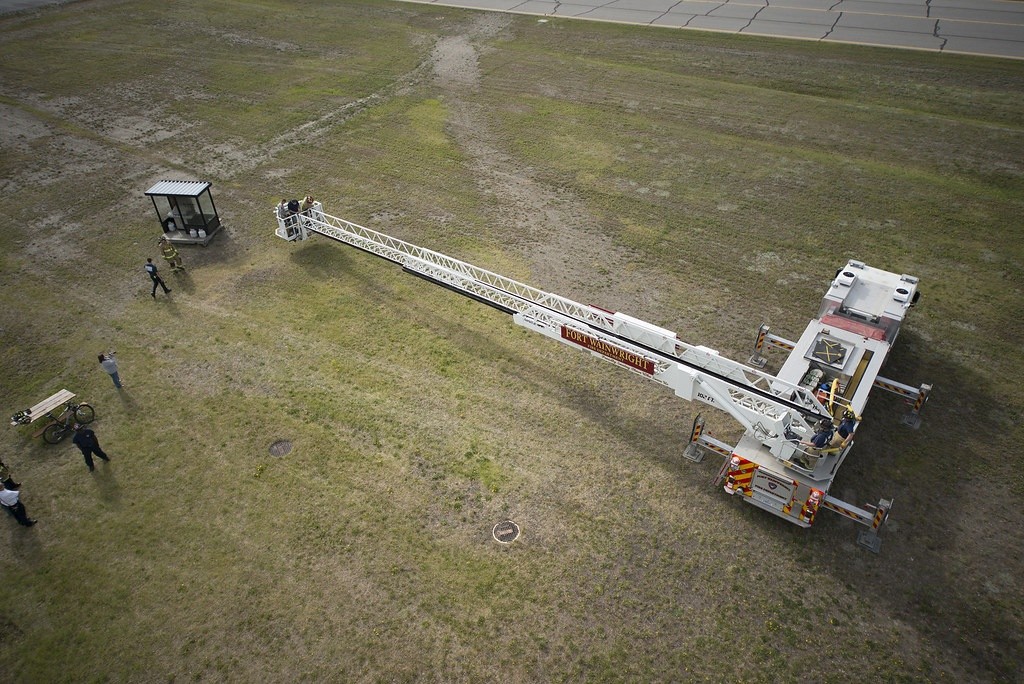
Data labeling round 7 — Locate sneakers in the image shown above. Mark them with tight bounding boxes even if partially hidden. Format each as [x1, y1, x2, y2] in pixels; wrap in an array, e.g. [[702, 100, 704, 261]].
[[151, 292, 155, 300], [114, 380, 124, 388], [165, 289, 171, 294], [170, 268, 178, 273], [177, 265, 185, 271]]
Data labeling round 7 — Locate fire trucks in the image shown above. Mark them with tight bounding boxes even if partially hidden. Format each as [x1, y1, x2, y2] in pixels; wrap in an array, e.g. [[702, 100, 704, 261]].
[[276, 191, 935, 532]]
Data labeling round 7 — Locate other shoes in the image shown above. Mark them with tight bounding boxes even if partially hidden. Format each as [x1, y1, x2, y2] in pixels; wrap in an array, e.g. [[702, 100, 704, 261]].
[[102, 456, 111, 461], [89, 463, 94, 471]]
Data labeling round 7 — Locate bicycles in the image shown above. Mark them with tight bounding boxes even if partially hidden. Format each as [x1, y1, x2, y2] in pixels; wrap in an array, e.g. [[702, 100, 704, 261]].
[[42, 399, 96, 445]]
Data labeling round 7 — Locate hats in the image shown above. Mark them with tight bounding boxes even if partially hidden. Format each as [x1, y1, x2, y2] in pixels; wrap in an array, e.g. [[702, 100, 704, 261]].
[[72, 424, 84, 430], [159, 236, 166, 240]]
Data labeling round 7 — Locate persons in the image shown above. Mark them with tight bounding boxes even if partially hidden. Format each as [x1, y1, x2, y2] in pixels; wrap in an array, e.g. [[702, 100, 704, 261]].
[[0, 460, 38, 527], [794, 423, 833, 470], [829, 408, 856, 456], [143, 258, 172, 299], [277, 195, 315, 235], [98, 352, 124, 388], [157, 236, 185, 272], [72, 424, 110, 472]]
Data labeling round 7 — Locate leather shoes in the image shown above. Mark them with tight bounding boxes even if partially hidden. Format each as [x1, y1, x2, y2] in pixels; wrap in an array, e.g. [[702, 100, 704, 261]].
[[10, 483, 21, 490], [19, 516, 37, 526]]
